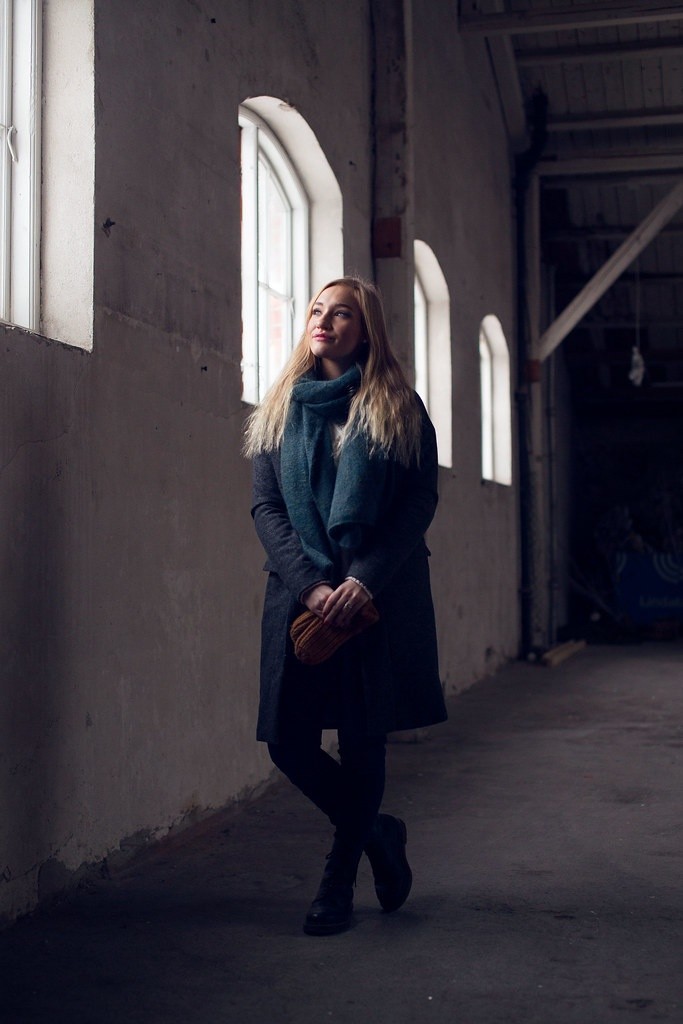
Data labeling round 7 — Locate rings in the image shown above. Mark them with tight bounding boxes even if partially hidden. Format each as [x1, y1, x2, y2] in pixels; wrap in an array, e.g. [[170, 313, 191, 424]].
[[344, 601, 352, 610]]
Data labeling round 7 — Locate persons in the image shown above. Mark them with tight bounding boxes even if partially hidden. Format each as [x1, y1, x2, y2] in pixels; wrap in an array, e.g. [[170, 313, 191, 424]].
[[246, 277, 449, 939]]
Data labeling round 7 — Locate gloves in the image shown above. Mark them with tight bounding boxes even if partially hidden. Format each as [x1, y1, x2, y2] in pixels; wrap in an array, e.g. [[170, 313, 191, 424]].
[[289, 597, 380, 665]]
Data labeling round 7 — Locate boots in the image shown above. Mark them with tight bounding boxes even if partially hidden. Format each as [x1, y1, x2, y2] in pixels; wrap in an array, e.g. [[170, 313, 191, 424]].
[[363, 814, 413, 911], [302, 850, 362, 937]]
